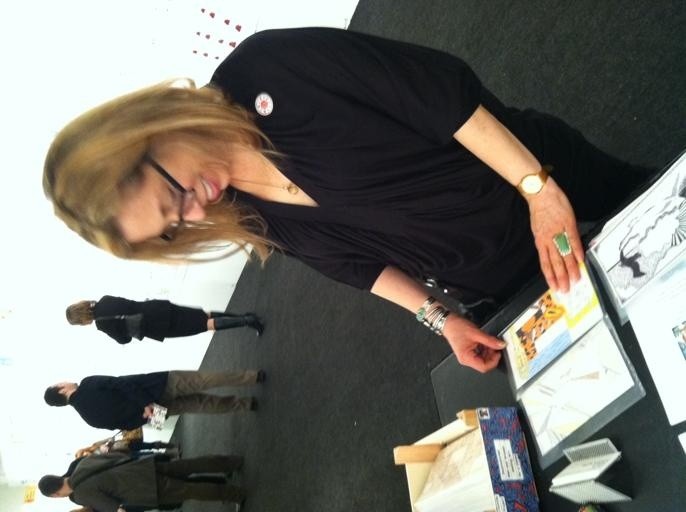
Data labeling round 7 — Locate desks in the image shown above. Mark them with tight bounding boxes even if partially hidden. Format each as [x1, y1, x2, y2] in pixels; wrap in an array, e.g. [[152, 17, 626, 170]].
[[429, 144, 686, 511]]
